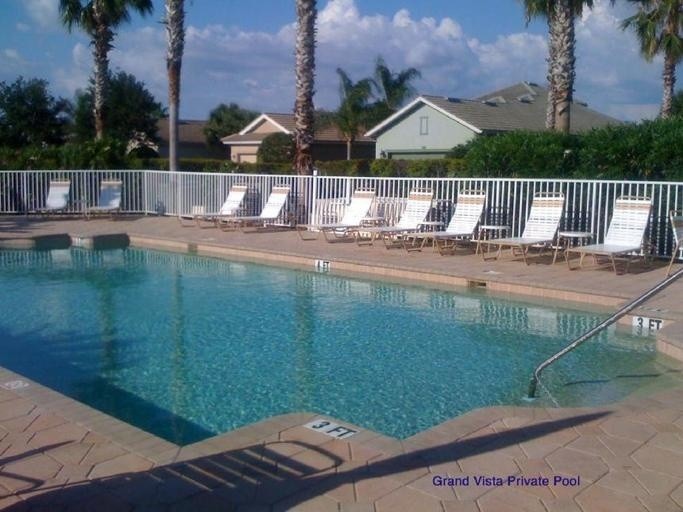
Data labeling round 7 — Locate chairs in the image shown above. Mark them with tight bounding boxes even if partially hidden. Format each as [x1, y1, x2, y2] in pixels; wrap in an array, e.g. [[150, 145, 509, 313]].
[[84, 179, 123, 221], [26, 179, 71, 223]]
